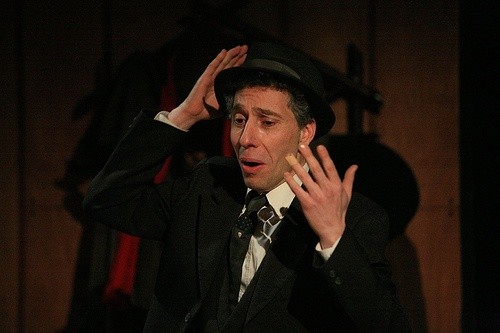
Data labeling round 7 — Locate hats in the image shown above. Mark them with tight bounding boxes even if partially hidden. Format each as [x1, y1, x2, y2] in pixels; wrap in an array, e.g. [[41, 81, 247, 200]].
[[213, 41, 336, 141]]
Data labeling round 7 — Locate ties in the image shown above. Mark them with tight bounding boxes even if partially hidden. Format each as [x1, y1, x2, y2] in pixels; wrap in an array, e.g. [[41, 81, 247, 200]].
[[218, 189, 268, 326]]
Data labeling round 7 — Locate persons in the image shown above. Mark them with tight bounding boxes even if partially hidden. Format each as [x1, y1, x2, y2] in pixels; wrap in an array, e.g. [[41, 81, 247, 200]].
[[81, 44, 410, 333]]
